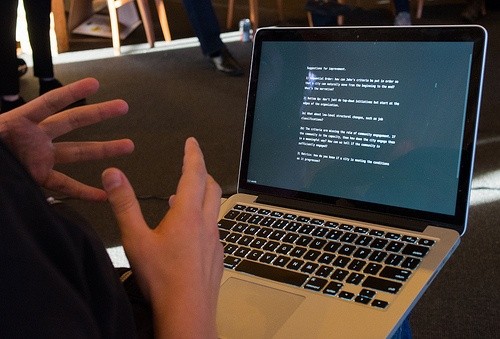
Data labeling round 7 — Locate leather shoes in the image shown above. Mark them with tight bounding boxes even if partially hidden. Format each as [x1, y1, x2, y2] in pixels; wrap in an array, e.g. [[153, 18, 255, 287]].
[[208, 57, 244, 76]]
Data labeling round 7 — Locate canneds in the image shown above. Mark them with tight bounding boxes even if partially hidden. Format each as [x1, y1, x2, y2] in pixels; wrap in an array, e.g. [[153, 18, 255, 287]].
[[239, 18, 250, 42]]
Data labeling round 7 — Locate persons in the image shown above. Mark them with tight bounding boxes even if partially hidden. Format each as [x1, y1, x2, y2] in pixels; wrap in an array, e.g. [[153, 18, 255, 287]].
[[0, 0, 87, 114], [0, 77, 224, 339]]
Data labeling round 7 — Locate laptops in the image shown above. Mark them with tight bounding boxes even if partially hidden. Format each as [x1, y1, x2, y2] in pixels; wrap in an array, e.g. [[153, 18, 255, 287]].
[[215, 25, 488, 339]]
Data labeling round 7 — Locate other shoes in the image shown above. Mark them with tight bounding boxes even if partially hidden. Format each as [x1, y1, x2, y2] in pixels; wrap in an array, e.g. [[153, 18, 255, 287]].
[[0, 95, 27, 113], [40, 79, 87, 113], [394, 12, 411, 26]]
